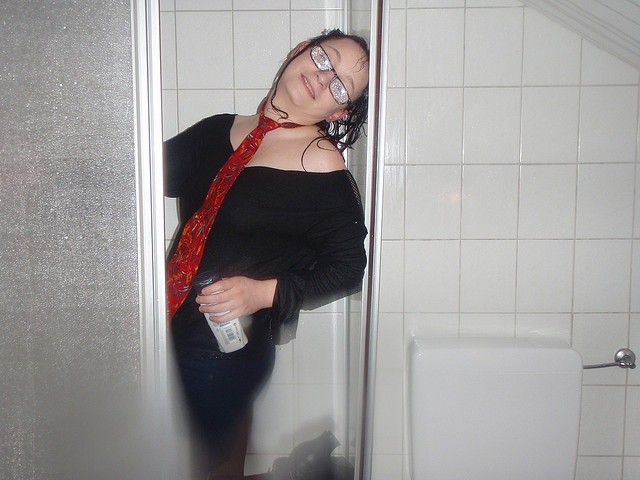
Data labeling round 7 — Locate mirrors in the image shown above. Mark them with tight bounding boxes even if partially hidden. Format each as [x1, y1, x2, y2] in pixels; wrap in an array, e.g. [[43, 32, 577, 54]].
[[130, 0, 390, 478]]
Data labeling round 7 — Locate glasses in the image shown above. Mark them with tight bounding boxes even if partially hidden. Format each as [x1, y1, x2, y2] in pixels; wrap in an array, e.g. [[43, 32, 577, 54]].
[[310, 44, 352, 108]]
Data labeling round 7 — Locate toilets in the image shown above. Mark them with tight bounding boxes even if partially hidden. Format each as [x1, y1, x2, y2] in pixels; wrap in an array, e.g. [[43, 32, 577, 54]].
[[402, 335, 584, 480]]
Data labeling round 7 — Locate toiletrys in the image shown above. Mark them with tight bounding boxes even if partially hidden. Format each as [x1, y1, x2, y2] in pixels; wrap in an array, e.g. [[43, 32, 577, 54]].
[[189, 270, 249, 353]]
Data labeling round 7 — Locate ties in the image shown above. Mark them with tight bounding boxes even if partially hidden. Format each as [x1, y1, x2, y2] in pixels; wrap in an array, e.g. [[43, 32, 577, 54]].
[[167, 99, 303, 320]]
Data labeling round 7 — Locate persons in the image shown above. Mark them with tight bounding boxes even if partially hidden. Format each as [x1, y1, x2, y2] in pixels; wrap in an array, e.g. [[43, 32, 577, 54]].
[[164, 29, 368, 479]]
